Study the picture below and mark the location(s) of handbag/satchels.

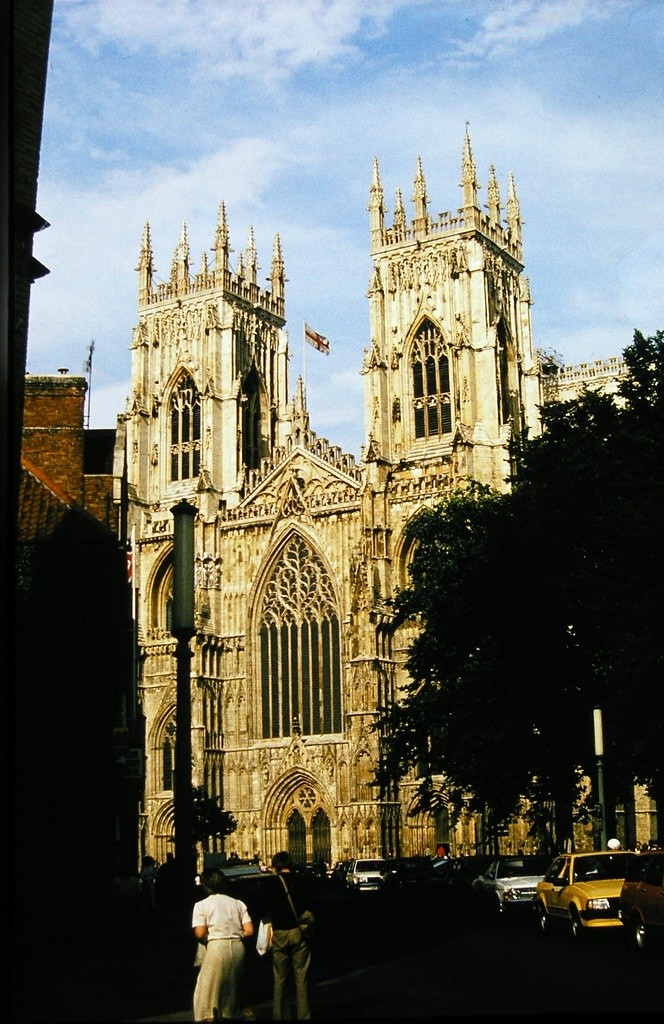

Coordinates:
[256,920,273,956]
[298,911,315,940]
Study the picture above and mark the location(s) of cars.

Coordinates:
[619,849,663,954]
[528,852,642,942]
[467,856,550,914]
[209,850,495,906]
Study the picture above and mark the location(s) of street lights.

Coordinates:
[169,497,198,887]
[592,705,608,855]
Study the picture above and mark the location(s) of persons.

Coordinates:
[261,850,319,1024]
[228,848,269,871]
[187,866,255,1024]
[436,845,446,858]
[422,844,433,858]
